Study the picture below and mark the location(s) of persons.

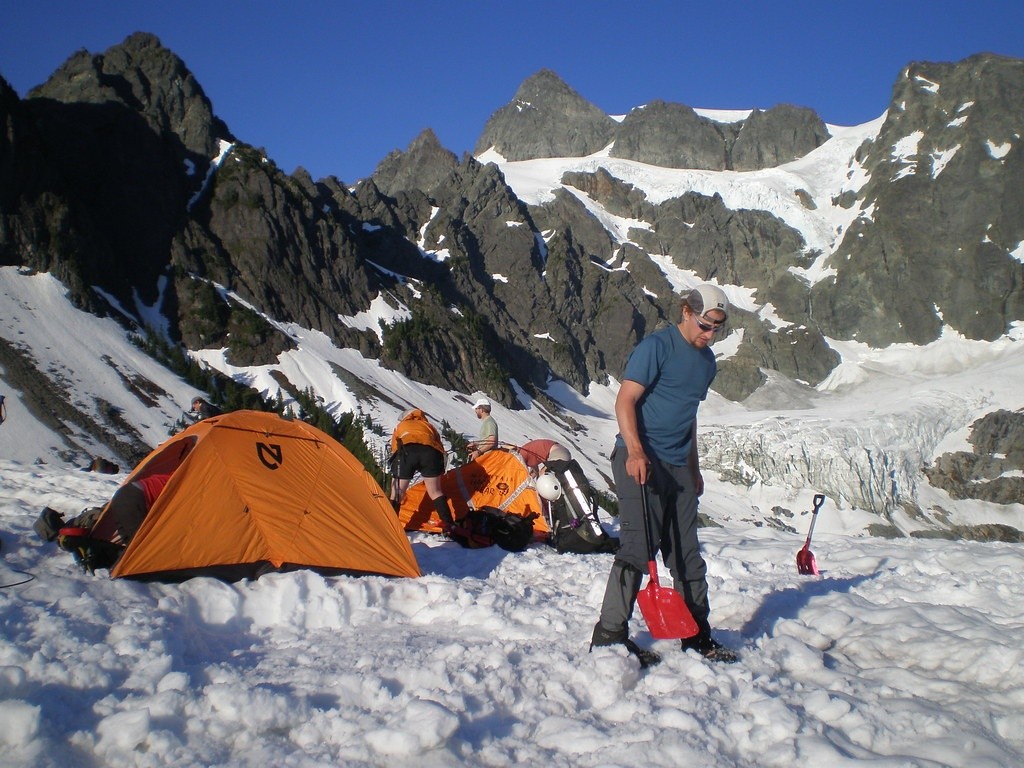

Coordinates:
[57,473,174,576]
[191,396,223,424]
[589,284,743,668]
[390,408,456,538]
[466,398,498,462]
[511,439,572,479]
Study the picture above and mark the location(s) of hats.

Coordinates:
[686,285,729,324]
[398,409,415,422]
[191,396,202,411]
[472,399,491,408]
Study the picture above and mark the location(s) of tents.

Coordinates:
[88,409,421,579]
[398,439,554,542]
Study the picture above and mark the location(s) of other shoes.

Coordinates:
[443,526,454,538]
[56,534,98,577]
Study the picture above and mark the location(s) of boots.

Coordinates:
[588,622,661,669]
[681,630,736,664]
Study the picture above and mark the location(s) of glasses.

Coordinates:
[691,312,720,332]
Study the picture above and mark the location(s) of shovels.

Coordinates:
[792,493,826,576]
[633,460,701,641]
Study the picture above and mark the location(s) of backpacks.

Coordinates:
[451,507,494,548]
[478,506,539,553]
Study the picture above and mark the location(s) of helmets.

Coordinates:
[536,471,562,502]
